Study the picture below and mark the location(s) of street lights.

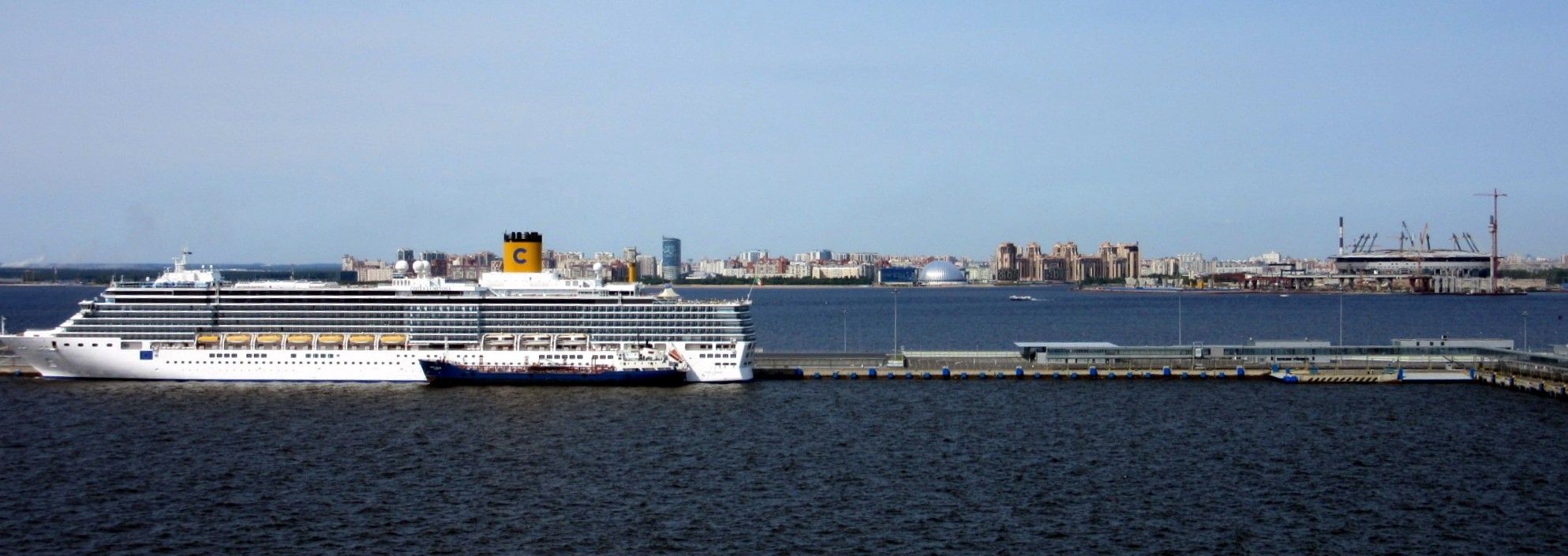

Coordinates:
[842,309,849,353]
[1336,288,1346,347]
[1522,310,1529,353]
[891,288,901,361]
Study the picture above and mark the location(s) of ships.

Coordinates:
[0,247,758,385]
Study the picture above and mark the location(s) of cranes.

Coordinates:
[1472,188,1508,280]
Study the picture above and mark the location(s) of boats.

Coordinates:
[350,335,374,344]
[657,287,680,301]
[416,357,688,388]
[226,334,250,344]
[317,334,343,344]
[288,335,313,344]
[1009,295,1031,301]
[197,336,219,343]
[258,335,282,344]
[382,335,406,346]
